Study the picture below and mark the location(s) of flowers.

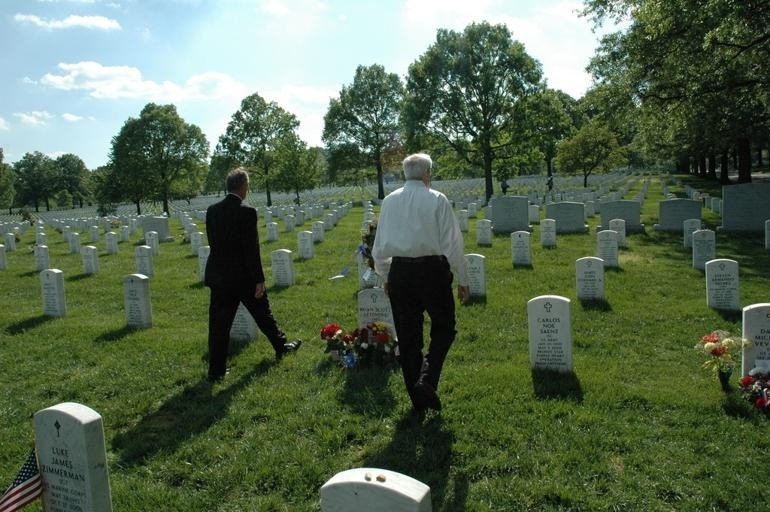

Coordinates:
[320,320,398,375]
[693,330,752,372]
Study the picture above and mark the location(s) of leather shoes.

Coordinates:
[206,367,224,384]
[275,339,301,362]
[412,377,442,412]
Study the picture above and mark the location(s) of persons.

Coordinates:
[204,168,302,382]
[371,153,471,412]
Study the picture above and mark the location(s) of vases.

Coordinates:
[717,369,732,388]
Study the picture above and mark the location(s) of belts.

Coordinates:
[391,254,445,263]
